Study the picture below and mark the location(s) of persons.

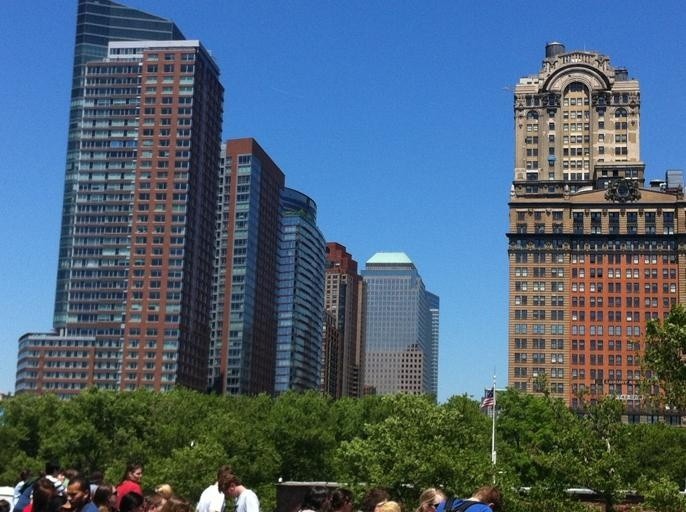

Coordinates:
[1,461,260,511]
[300,485,498,512]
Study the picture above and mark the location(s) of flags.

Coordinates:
[481,387,494,408]
